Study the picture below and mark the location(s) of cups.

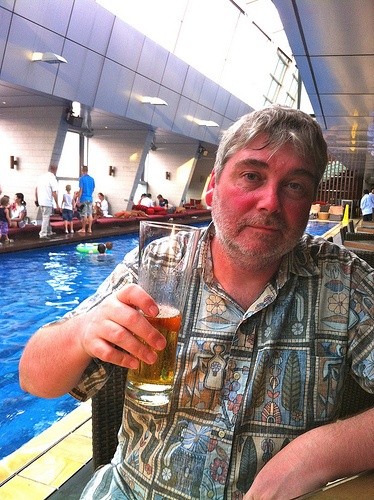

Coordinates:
[120,222,202,414]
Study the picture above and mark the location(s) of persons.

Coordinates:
[359,188,374,221]
[17,104,374,500]
[0,193,27,245]
[77,165,95,233]
[105,242,113,254]
[97,244,106,255]
[61,184,168,233]
[34,165,61,241]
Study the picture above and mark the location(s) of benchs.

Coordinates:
[0,208,220,238]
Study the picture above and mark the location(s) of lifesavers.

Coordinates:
[76,243,107,253]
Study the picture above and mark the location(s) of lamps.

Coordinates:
[166,171,171,180]
[10,156,17,169]
[198,144,209,161]
[66,102,86,128]
[33,51,68,66]
[142,95,169,106]
[207,121,217,128]
[109,165,114,176]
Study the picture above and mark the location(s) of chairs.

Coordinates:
[329,205,344,220]
[308,204,321,218]
[91,368,372,472]
[347,249,374,268]
[340,220,374,244]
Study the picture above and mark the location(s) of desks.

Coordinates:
[295,473,374,500]
[318,211,329,219]
[360,220,374,227]
[356,227,374,234]
[344,240,374,251]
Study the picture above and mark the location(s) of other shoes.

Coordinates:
[0,242,5,246]
[5,239,16,243]
[65,230,74,234]
[40,232,56,239]
[76,230,91,234]
